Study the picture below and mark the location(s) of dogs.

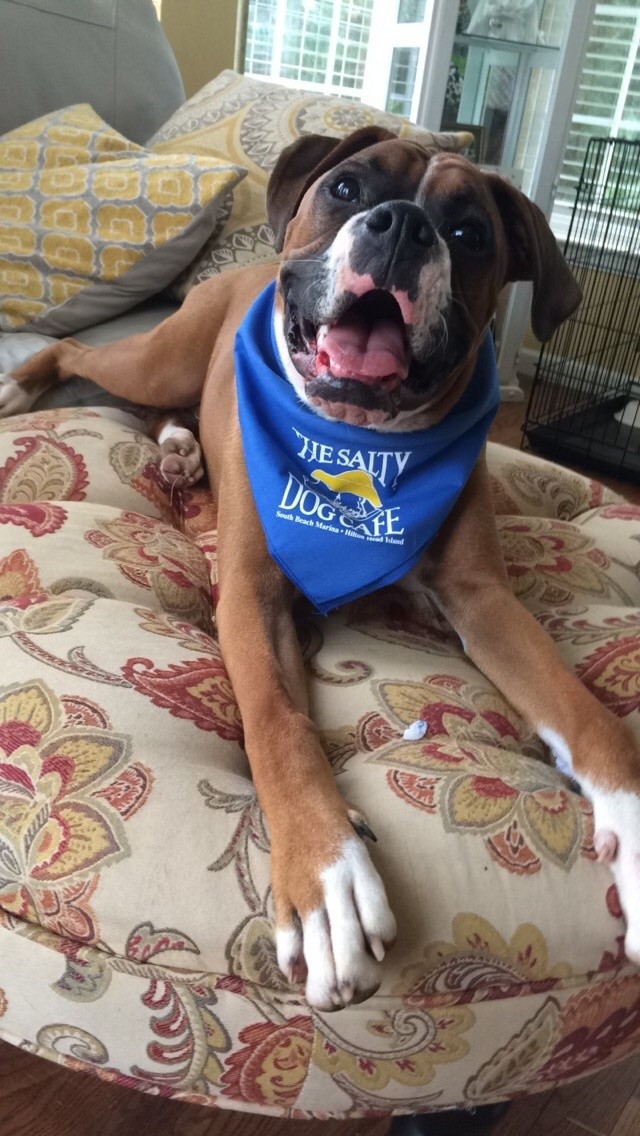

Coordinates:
[0,115,640,1018]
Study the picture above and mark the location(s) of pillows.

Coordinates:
[0,103,249,335]
[141,67,475,305]
